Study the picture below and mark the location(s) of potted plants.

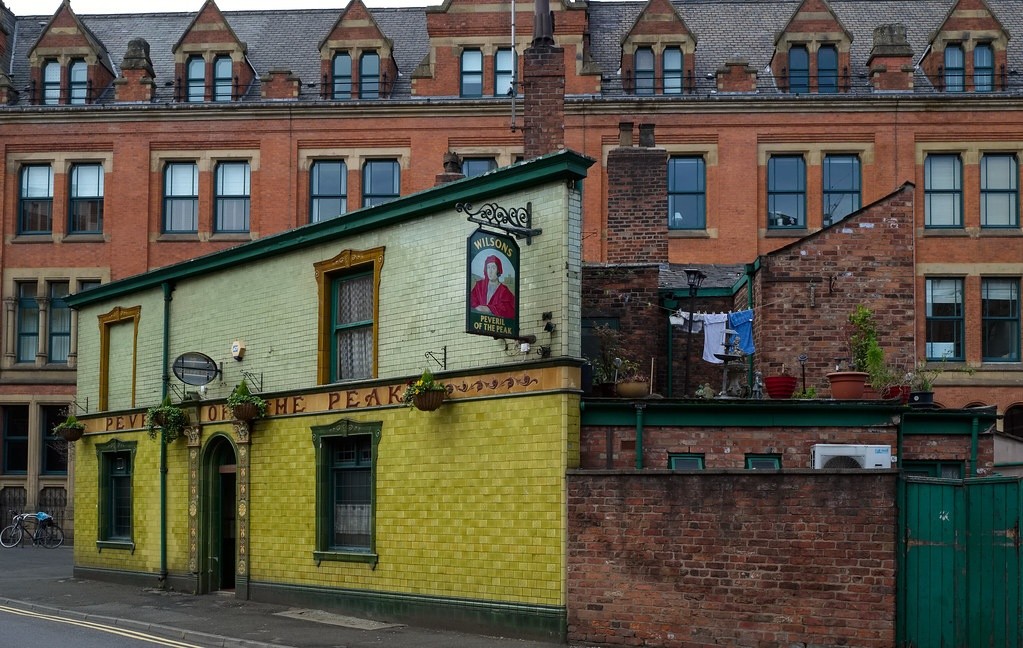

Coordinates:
[763,363,798,398]
[53,413,86,442]
[881,367,912,404]
[148,396,190,444]
[863,335,884,399]
[910,348,976,408]
[593,322,625,397]
[617,359,650,397]
[581,355,599,397]
[828,303,879,401]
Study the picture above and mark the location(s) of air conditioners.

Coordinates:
[810,442,891,469]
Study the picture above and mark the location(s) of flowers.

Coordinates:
[226,379,268,418]
[400,369,445,411]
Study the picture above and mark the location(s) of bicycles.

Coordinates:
[0,509,64,549]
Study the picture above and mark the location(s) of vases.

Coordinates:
[413,389,445,411]
[233,404,258,422]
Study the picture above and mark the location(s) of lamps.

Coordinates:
[672,211,683,227]
[694,274,707,291]
[684,268,699,289]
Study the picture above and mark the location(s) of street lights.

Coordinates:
[684,265,707,397]
